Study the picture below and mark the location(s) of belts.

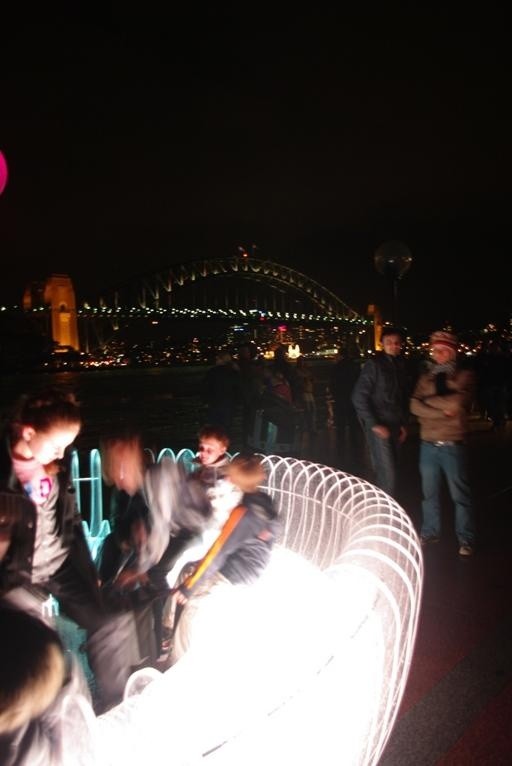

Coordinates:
[426,440,464,447]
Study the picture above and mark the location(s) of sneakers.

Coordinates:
[419,535,441,545]
[458,542,472,557]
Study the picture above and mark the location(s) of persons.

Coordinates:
[0,385,276,765]
[353,324,415,496]
[196,346,365,462]
[409,329,479,558]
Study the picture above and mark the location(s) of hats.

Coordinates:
[429,327,460,359]
